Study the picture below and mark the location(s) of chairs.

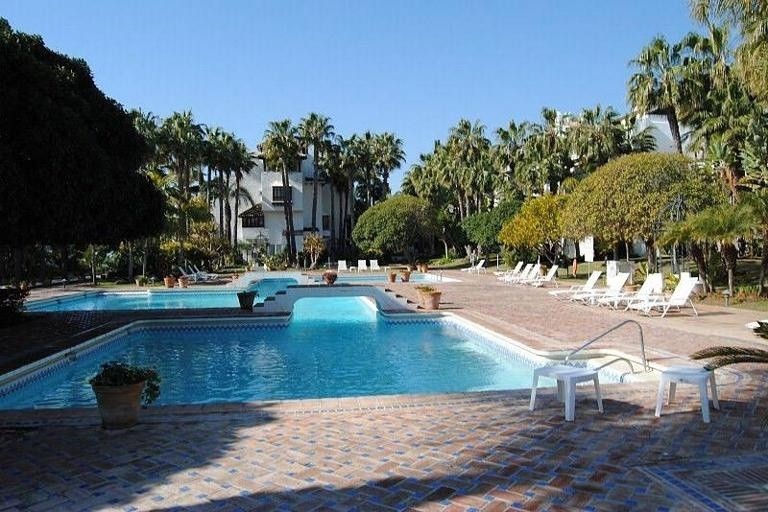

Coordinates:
[549,270,700,319]
[468,259,486,275]
[337,259,348,274]
[357,259,367,274]
[370,259,380,272]
[178,263,220,284]
[493,261,559,289]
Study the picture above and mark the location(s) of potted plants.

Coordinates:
[387,272,396,282]
[400,270,411,281]
[135,276,148,287]
[88,357,162,428]
[415,283,441,310]
[237,290,259,312]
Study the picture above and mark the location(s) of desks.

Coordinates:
[350,266,356,272]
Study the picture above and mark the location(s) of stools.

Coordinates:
[655,366,720,425]
[529,365,605,421]
